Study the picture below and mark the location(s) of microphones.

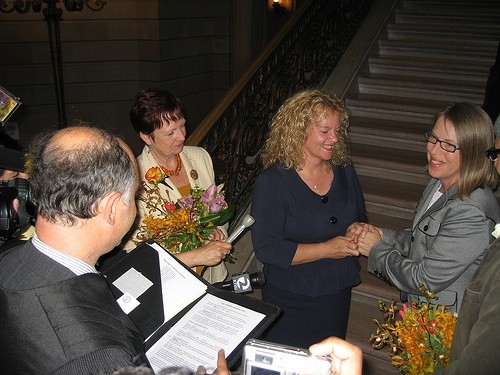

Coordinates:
[212,272,266,294]
[226,215,255,244]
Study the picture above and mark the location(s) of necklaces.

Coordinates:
[152,153,182,176]
[300,168,329,193]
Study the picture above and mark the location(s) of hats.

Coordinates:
[0,133,26,172]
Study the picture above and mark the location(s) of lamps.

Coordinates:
[272,0,286,17]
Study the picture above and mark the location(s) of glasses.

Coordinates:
[485,147,500,162]
[424,131,459,152]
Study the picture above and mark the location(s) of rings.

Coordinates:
[224,249,228,254]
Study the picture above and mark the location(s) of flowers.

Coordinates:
[129,166,237,265]
[368,284,457,375]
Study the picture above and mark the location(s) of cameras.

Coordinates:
[242,339,332,375]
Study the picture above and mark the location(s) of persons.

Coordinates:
[432,116,500,375]
[0,88,233,375]
[252,89,368,350]
[346,103,495,366]
[309,337,363,375]
